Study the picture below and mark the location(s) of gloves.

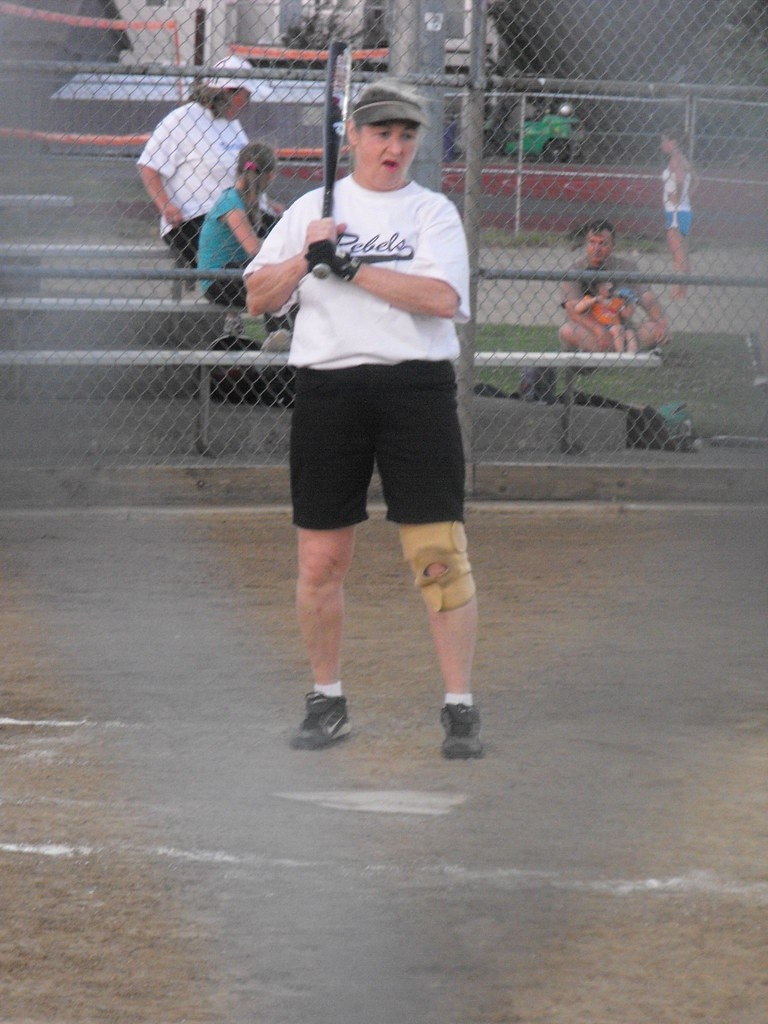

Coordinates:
[305,239,362,282]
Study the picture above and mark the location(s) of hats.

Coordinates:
[350,80,434,131]
[202,55,256,93]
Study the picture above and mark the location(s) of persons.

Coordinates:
[136,53,287,269]
[573,282,640,354]
[197,140,300,352]
[661,128,701,304]
[558,220,670,354]
[243,83,485,758]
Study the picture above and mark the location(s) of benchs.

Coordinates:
[0,194,661,460]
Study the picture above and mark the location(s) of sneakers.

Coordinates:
[291,692,352,750]
[440,703,485,759]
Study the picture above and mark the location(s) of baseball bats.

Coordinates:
[312,38,352,281]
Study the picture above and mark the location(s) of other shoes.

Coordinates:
[225,318,245,334]
[259,329,291,352]
